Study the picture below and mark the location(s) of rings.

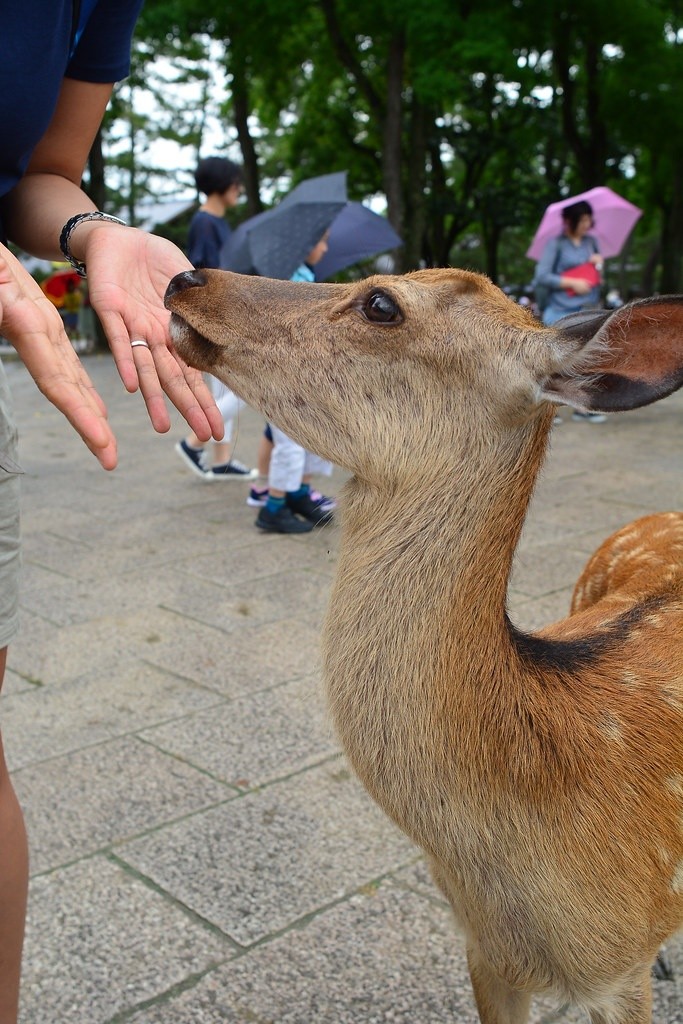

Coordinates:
[130,341,148,347]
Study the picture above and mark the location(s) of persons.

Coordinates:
[519,286,539,319]
[0,0,223,1024]
[534,201,607,423]
[247,422,336,511]
[175,156,258,480]
[605,289,623,310]
[253,228,332,535]
[63,279,81,352]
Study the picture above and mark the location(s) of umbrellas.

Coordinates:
[525,186,641,262]
[222,200,402,282]
[220,172,347,282]
[39,269,82,308]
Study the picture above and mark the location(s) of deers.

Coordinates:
[163,267,683,1024]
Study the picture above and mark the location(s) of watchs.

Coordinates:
[594,263,603,270]
[59,211,126,278]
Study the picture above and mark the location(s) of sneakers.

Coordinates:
[212,459,260,480]
[290,495,334,526]
[310,491,337,511]
[256,506,313,533]
[573,411,606,423]
[174,439,213,481]
[554,415,563,424]
[246,485,270,507]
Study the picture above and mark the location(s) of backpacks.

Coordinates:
[533,235,599,312]
